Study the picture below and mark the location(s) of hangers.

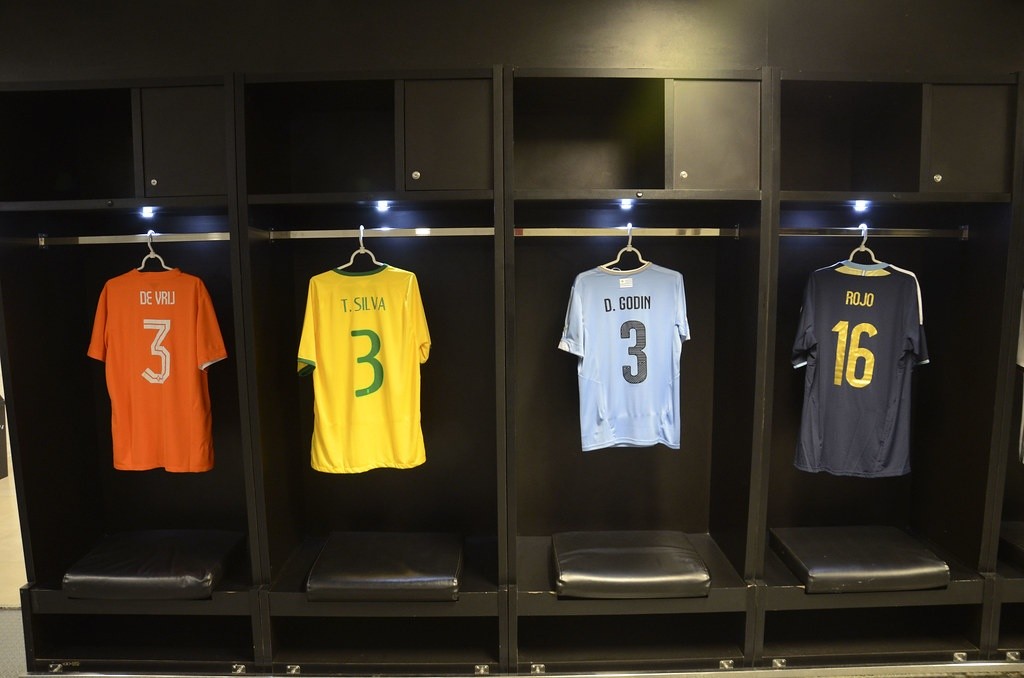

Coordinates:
[135,229,175,275]
[599,221,652,276]
[335,224,387,274]
[842,223,889,266]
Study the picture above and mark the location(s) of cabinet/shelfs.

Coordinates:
[6,70,1022,675]
